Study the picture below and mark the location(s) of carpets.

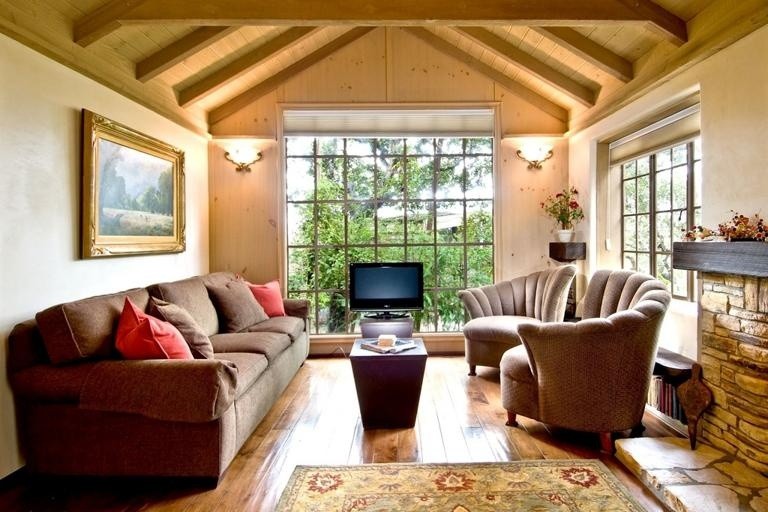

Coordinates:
[275,458,652,512]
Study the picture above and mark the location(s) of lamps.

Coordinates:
[213,137,272,173]
[505,135,561,171]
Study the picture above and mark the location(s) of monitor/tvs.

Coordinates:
[350,262,424,319]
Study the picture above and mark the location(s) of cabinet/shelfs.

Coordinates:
[360,317,412,336]
[644,347,697,440]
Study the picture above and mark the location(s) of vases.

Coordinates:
[731,237,756,242]
[556,230,574,242]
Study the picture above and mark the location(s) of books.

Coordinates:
[647,371,689,424]
[360,332,414,354]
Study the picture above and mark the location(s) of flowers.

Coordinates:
[677,211,768,245]
[539,186,585,230]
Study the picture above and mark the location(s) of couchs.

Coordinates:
[499,269,672,457]
[457,265,578,376]
[6,271,313,489]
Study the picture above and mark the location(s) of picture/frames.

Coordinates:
[78,108,186,261]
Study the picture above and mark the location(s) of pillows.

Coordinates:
[113,297,194,361]
[204,280,270,332]
[248,280,285,318]
[145,299,214,361]
